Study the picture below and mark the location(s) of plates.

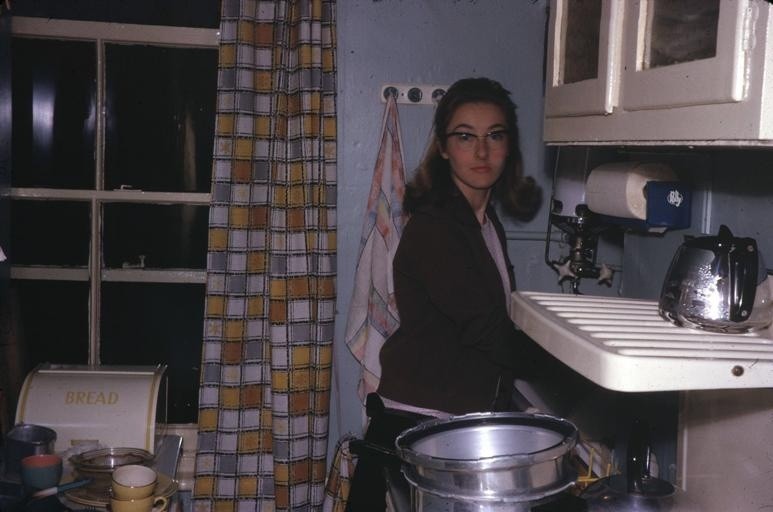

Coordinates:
[64,471,181,506]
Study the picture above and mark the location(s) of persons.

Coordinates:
[342,77,547,511]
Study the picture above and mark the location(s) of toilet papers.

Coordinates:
[584,161,677,220]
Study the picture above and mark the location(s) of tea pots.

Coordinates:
[572,416,700,512]
[657,226,772,335]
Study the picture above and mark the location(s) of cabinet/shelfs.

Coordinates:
[542,0,773,147]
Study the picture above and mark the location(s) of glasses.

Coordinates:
[446,129,516,150]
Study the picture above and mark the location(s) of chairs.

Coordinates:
[15,362,183,480]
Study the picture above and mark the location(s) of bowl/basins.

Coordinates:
[66,445,155,494]
[19,453,61,486]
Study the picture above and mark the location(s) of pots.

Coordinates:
[393,410,582,512]
[2,423,58,469]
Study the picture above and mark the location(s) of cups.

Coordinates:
[110,463,157,501]
[108,492,167,511]
